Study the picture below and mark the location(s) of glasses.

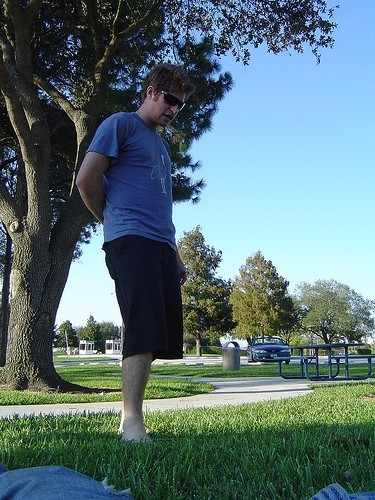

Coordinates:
[160,90,185,111]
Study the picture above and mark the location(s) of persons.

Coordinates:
[76,63,197,444]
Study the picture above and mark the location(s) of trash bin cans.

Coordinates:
[221,341,240,372]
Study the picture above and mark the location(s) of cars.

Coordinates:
[246,335,290,364]
